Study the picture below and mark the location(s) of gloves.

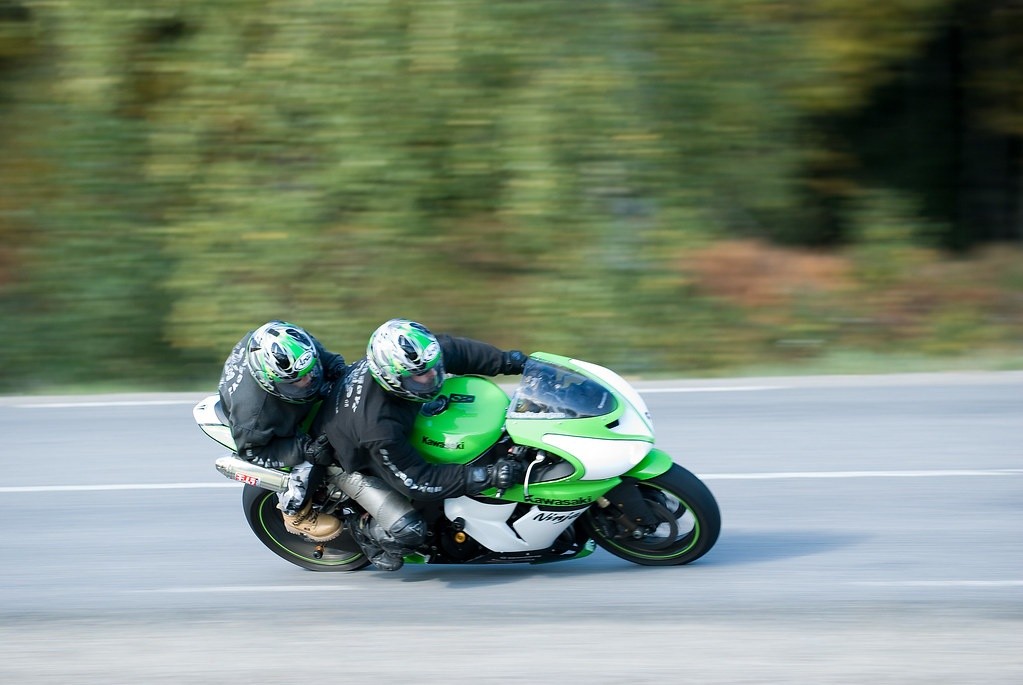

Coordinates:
[345,361,357,373]
[466,461,521,494]
[302,433,334,466]
[502,350,557,381]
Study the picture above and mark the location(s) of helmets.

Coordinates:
[246,319,324,406]
[366,318,445,403]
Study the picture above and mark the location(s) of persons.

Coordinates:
[218,320,349,544]
[323,318,528,571]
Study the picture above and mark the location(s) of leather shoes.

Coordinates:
[276,501,344,542]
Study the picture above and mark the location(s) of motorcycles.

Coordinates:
[193,350,721,573]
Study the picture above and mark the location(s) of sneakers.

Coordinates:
[345,513,405,571]
